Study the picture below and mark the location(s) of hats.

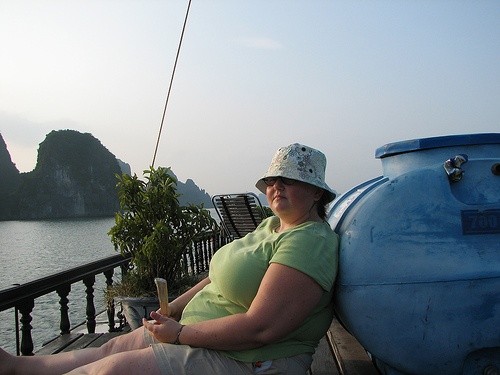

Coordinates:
[254,143,337,206]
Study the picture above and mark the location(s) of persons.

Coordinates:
[0,142,340,375]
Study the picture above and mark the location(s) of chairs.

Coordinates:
[210,193,268,238]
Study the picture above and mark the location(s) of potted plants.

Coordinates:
[103,166,221,329]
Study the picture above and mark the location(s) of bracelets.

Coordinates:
[175,324,185,345]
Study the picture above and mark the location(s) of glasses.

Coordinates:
[262,177,297,186]
